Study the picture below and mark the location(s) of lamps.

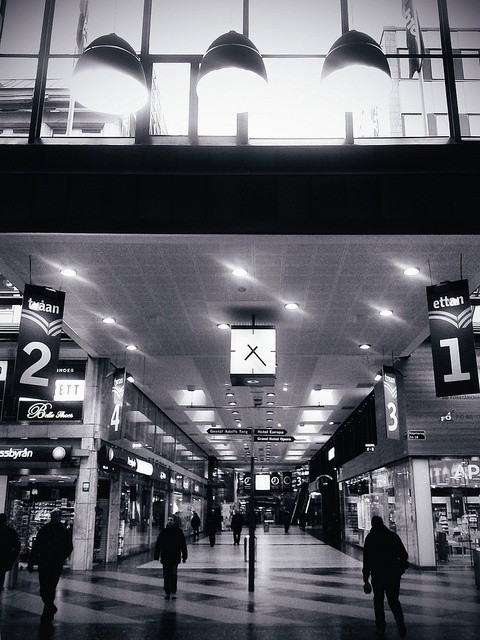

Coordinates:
[319,29,392,114]
[69,33,149,115]
[374,371,384,381]
[194,30,269,114]
[109,361,135,385]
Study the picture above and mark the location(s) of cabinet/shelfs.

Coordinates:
[9,499,31,558]
[28,499,75,552]
[461,496,480,532]
[431,496,452,541]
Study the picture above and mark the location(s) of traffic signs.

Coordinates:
[254,436,296,442]
[207,428,251,434]
[254,429,287,435]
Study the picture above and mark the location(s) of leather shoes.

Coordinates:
[165,593,170,600]
[172,593,176,600]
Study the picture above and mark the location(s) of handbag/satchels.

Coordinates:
[364,581,371,594]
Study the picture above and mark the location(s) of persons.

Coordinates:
[1,513,21,595]
[362,515,408,637]
[27,509,73,628]
[283,510,290,533]
[205,513,218,547]
[175,512,181,528]
[154,515,187,599]
[231,510,243,545]
[191,513,201,542]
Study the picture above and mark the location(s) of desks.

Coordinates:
[453,538,473,559]
